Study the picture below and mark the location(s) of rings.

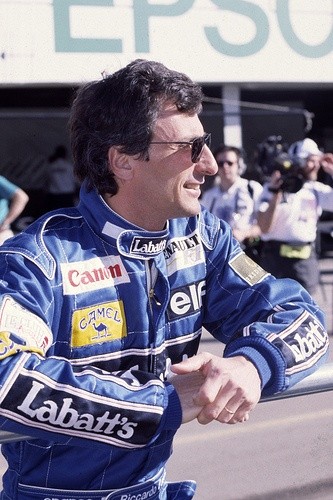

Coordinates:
[225,407,235,415]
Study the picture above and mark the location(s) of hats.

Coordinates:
[289,138,321,169]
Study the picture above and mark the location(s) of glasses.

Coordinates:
[217,160,239,168]
[148,133,211,163]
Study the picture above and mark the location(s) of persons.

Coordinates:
[38,146,81,208]
[0,58,329,500]
[0,176,29,245]
[200,145,263,251]
[251,139,333,295]
